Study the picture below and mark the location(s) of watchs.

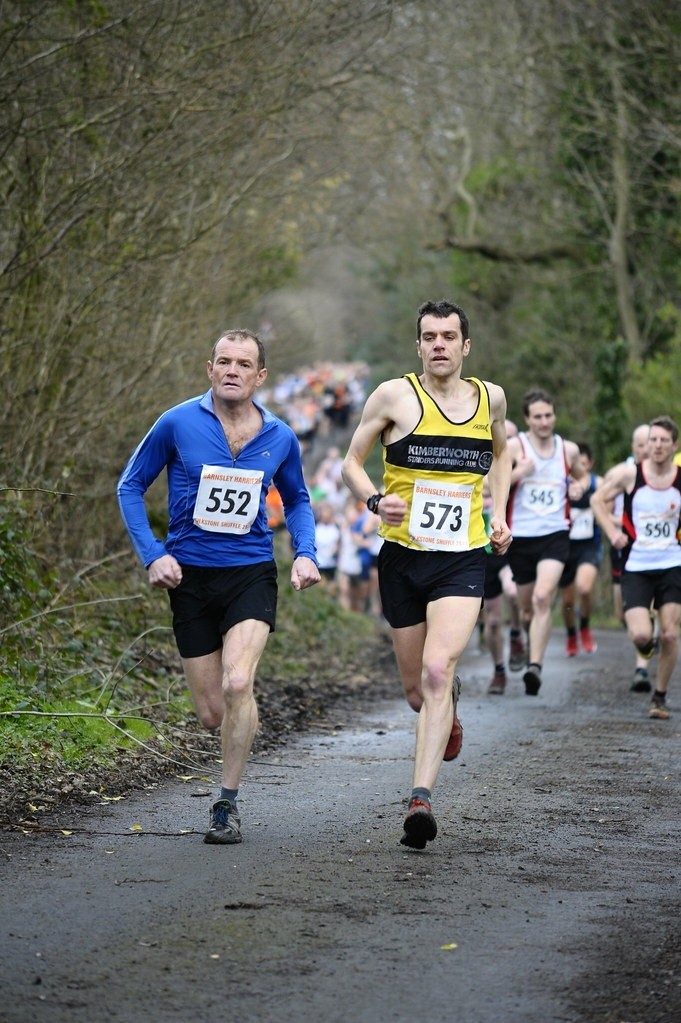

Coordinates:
[367,494,385,515]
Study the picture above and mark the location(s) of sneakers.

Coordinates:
[400,796,438,849]
[440,675,463,761]
[205,798,241,845]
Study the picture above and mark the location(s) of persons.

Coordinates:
[477,389,681,718]
[116,329,322,844]
[257,360,370,472]
[341,300,512,850]
[266,443,384,617]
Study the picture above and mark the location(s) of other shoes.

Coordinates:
[650,701,669,719]
[567,631,579,655]
[523,671,541,695]
[489,673,506,694]
[580,628,597,655]
[631,668,651,692]
[508,632,526,671]
